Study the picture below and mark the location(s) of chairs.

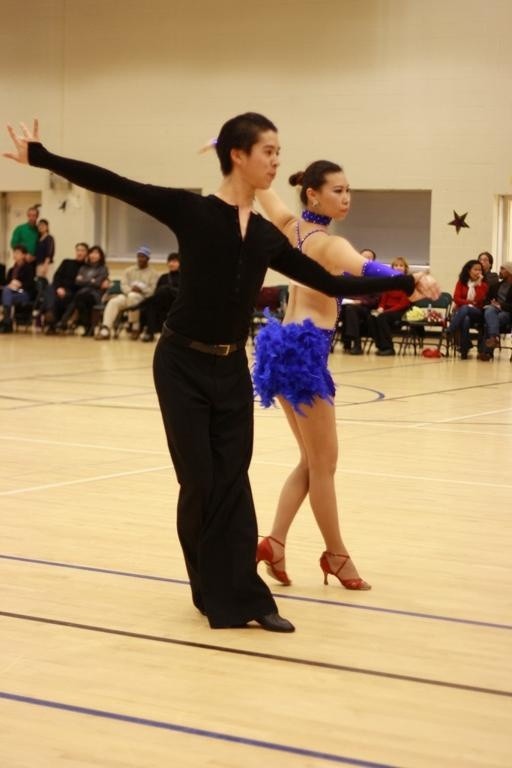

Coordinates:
[0,265,512,361]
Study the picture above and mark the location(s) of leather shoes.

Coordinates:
[257,613,296,633]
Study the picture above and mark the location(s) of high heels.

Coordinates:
[319,552,368,590]
[256,535,292,586]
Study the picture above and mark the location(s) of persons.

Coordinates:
[0,208,110,337]
[193,130,441,592]
[98,247,181,341]
[0,111,425,634]
[341,250,448,355]
[447,252,512,363]
